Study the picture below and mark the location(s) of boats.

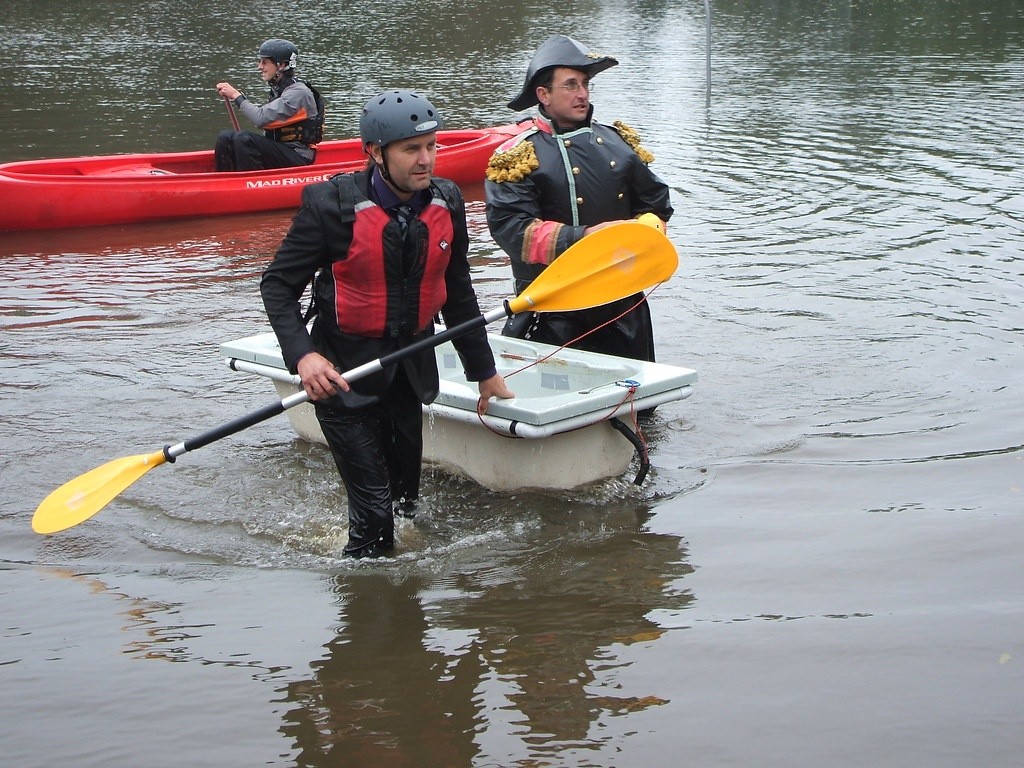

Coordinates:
[0,115,539,234]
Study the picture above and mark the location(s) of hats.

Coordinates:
[506,33,619,112]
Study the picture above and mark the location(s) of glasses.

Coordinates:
[545,81,595,92]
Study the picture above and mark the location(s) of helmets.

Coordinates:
[257,39,298,64]
[360,90,442,153]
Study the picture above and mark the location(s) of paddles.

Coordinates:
[31,222,678,534]
[223,95,240,132]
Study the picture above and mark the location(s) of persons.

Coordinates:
[215,39,325,172]
[484,34,674,364]
[261,90,515,558]
